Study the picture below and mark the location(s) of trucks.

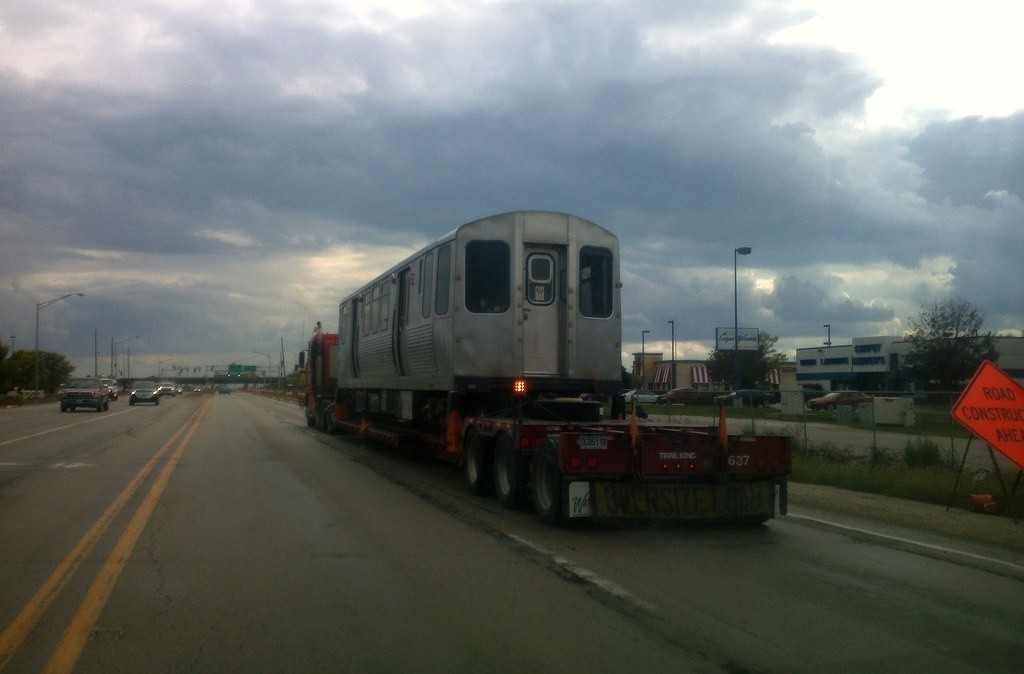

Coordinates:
[296,335,794,532]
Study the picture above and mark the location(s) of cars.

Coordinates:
[157,382,176,398]
[714,389,777,410]
[806,390,874,413]
[658,387,699,405]
[174,384,183,396]
[621,390,659,403]
[100,379,120,401]
[192,386,201,392]
[129,381,159,407]
[59,377,109,411]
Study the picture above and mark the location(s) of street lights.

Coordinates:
[115,335,138,379]
[733,246,752,389]
[32,293,84,405]
